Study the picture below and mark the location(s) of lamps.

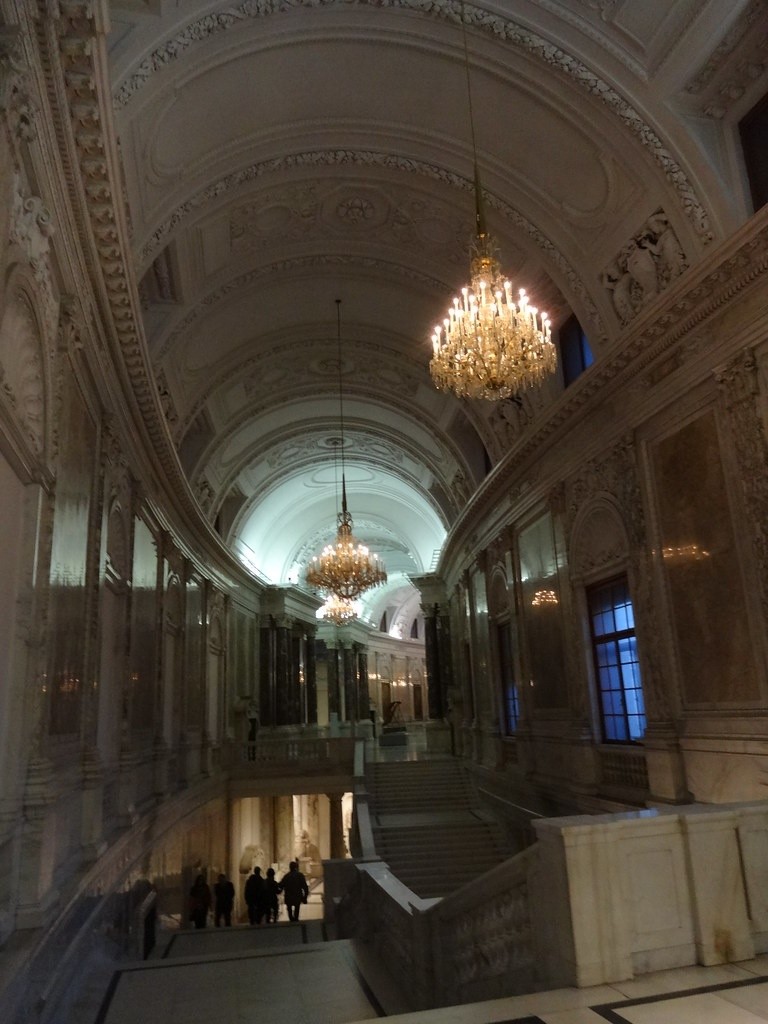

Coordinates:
[303,300,390,627]
[428,1,556,400]
[530,524,558,608]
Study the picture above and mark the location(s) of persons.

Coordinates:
[369,697,376,724]
[244,866,284,925]
[213,874,234,927]
[189,875,212,929]
[489,390,535,454]
[279,862,308,921]
[602,215,688,321]
